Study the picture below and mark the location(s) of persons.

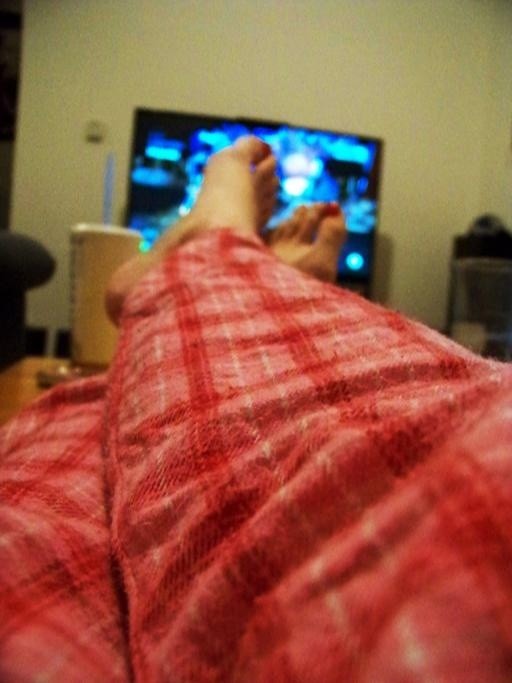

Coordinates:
[1,132,511,681]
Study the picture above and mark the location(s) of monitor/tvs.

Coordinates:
[123,106,383,284]
